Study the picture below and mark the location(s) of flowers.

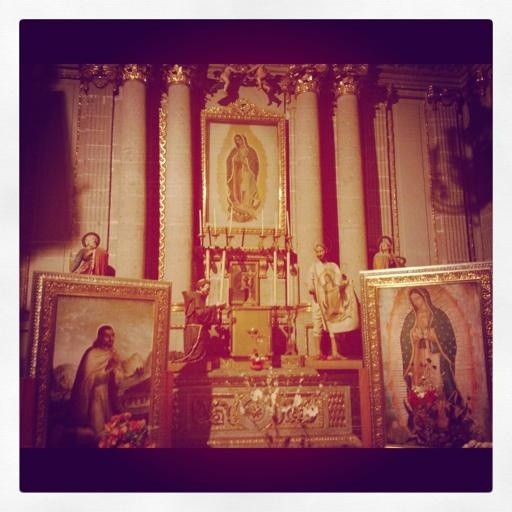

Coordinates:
[99,414,157,448]
[404,373,474,449]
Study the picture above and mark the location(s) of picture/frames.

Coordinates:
[19,270,172,448]
[201,97,287,235]
[360,261,493,449]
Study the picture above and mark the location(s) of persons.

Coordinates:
[68,325,125,440]
[70,232,107,277]
[309,242,344,361]
[234,264,254,290]
[322,272,343,318]
[400,286,464,415]
[182,279,227,364]
[372,236,399,271]
[226,132,259,223]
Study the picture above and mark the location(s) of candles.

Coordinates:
[220,251,225,302]
[205,250,209,306]
[261,210,264,235]
[274,211,278,236]
[213,208,216,233]
[274,251,277,305]
[230,206,232,235]
[199,211,202,235]
[287,252,290,306]
